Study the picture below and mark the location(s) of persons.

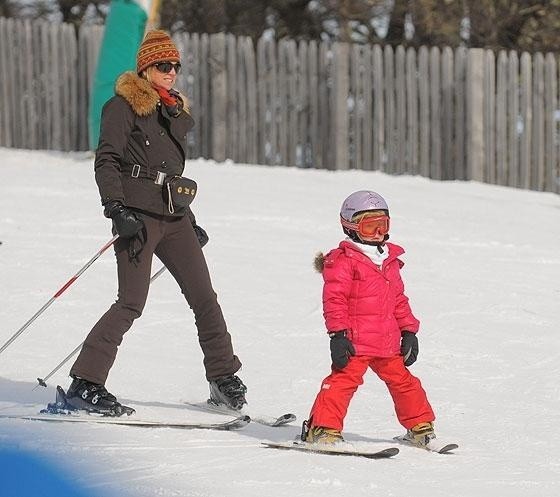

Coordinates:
[64,30,248,412]
[301,191,446,452]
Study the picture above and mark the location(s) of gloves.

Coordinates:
[103,200,143,240]
[330,330,356,366]
[193,225,209,249]
[399,330,419,366]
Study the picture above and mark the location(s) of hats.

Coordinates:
[136,30,181,74]
[340,190,390,234]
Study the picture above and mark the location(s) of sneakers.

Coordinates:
[210,374,248,411]
[306,425,344,447]
[408,422,436,444]
[54,378,136,417]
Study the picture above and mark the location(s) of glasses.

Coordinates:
[155,62,181,74]
[358,216,391,237]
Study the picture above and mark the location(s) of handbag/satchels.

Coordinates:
[163,175,197,207]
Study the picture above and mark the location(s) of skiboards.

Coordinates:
[1,394,295,430]
[262,432,457,458]
